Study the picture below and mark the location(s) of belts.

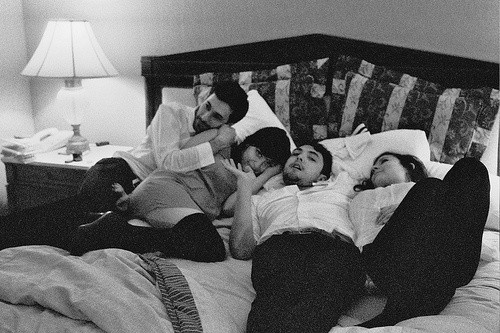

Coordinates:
[284,227,352,244]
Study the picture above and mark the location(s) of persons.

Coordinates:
[221,141,357,333]
[71,127,291,263]
[76,80,250,224]
[347,153,491,328]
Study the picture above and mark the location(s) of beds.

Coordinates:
[0,34,500,333]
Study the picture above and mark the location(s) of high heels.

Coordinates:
[66,211,123,247]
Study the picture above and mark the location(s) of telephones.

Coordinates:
[29,127,74,156]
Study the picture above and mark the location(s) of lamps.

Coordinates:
[21,19,121,159]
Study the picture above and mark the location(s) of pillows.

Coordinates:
[193,57,332,144]
[315,129,431,177]
[329,55,500,163]
[431,161,500,231]
[232,89,298,157]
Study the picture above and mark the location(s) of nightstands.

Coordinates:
[6,144,137,213]
